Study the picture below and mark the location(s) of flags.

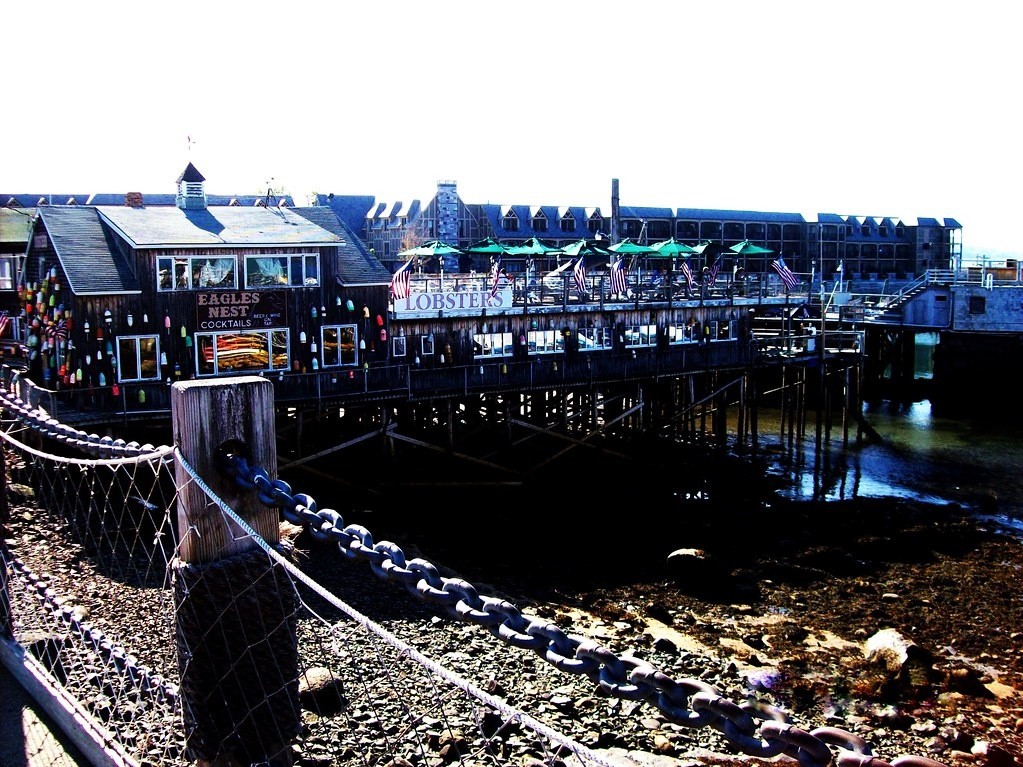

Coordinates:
[680,263,694,289]
[490,254,504,298]
[771,256,796,291]
[608,259,626,295]
[391,257,416,299]
[574,255,587,292]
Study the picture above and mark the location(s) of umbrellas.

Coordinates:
[395,239,464,272]
[463,238,513,271]
[604,238,660,268]
[694,240,736,271]
[730,238,775,270]
[649,235,696,270]
[505,236,566,272]
[560,237,610,275]
